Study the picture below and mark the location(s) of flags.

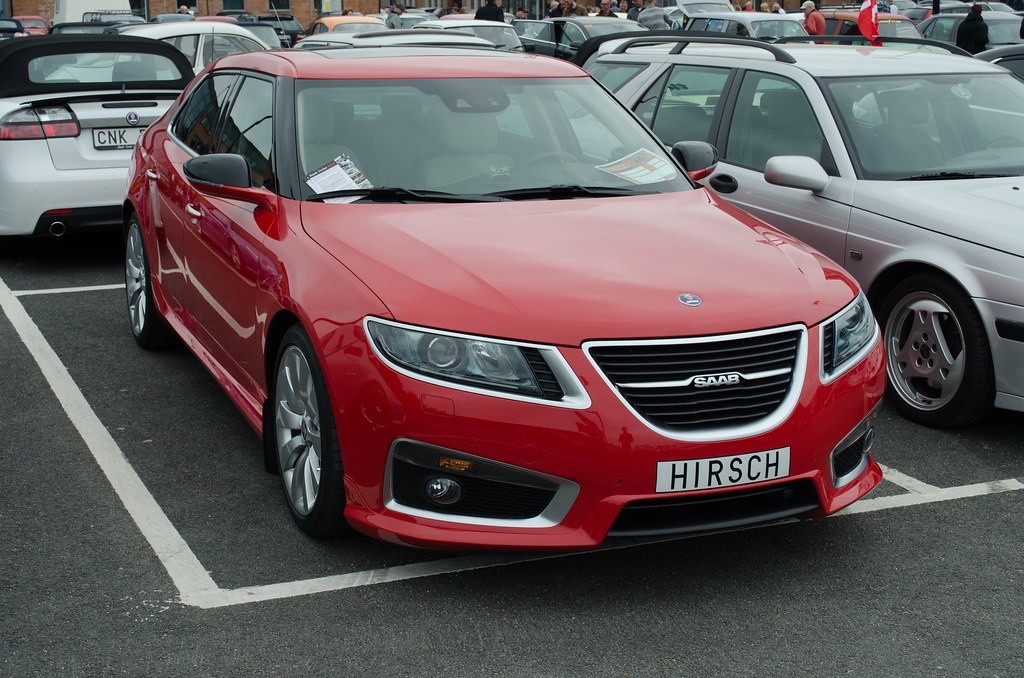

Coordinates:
[856,0,882,46]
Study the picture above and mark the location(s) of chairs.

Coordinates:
[331,101,374,179]
[653,106,707,146]
[190,35,213,67]
[870,93,944,169]
[373,96,442,188]
[417,106,517,189]
[302,111,366,189]
[111,61,157,81]
[745,91,821,169]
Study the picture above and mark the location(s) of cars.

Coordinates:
[120,41,885,557]
[566,38,1024,433]
[0,0,1024,242]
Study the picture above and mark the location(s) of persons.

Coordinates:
[800,1,826,48]
[385,4,408,32]
[474,0,788,27]
[341,9,354,18]
[1020,10,1024,42]
[448,6,469,17]
[864,0,901,16]
[957,4,989,55]
[178,6,191,16]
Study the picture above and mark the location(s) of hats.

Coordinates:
[800,1,815,9]
[395,4,405,13]
